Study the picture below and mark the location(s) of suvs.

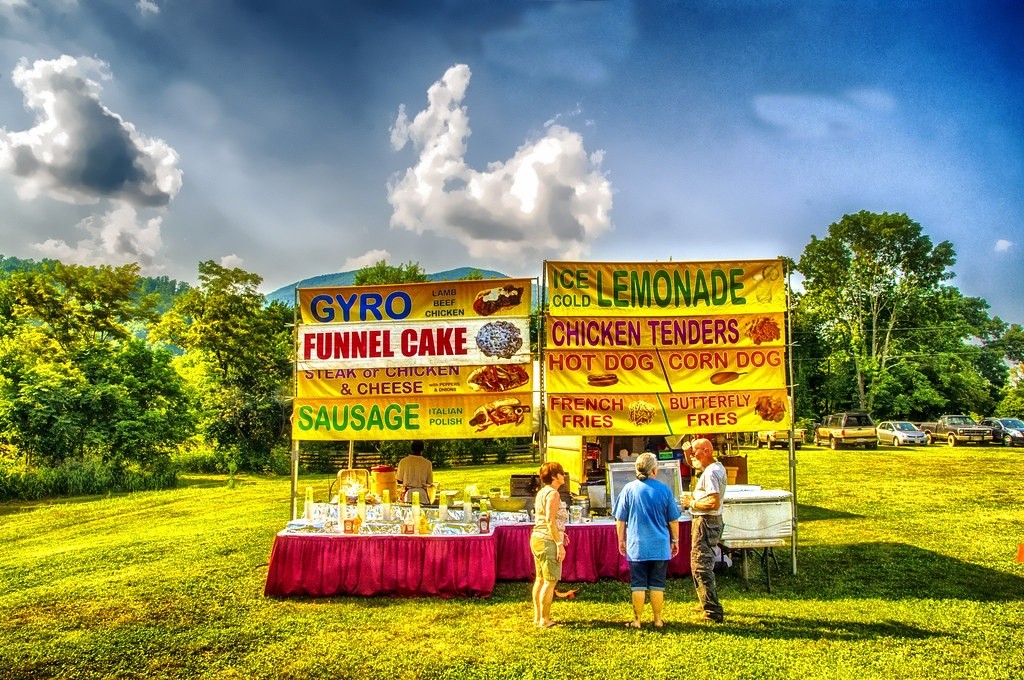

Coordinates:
[978,419,1024,448]
[812,412,877,451]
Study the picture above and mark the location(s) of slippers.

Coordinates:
[625,621,641,630]
[653,620,665,628]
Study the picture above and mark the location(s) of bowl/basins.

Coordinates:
[490,497,527,511]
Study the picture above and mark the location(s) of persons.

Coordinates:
[530,462,570,629]
[680,438,727,623]
[395,440,433,505]
[613,452,681,630]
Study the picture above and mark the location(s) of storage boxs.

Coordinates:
[719,491,792,541]
[508,427,768,502]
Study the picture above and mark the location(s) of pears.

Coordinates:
[710,372,748,385]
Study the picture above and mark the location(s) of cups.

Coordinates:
[570,506,582,524]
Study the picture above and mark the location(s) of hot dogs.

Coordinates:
[587,374,619,386]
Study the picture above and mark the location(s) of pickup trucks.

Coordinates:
[920,415,994,447]
[757,429,804,450]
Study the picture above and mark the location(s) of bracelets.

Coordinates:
[672,539,679,542]
[690,500,697,510]
[556,541,563,546]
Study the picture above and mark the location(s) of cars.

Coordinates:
[876,421,929,447]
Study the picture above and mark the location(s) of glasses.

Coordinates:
[690,447,705,456]
[556,471,564,476]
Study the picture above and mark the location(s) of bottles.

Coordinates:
[404,512,414,534]
[344,514,353,534]
[353,514,362,534]
[479,513,489,534]
[419,514,427,534]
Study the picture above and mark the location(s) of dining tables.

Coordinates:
[267,503,693,598]
